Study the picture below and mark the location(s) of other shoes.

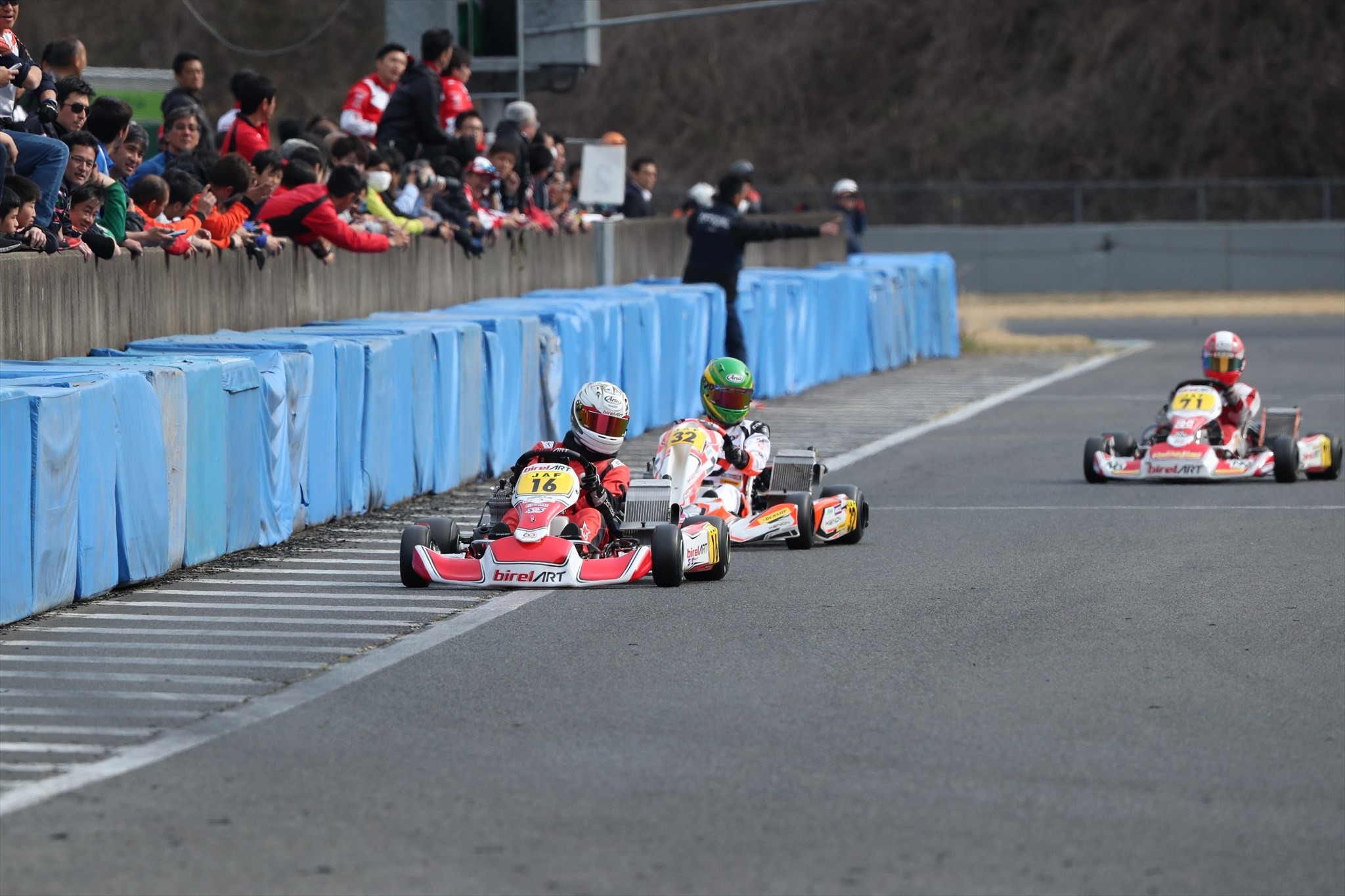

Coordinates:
[487,521,511,540]
[699,490,718,516]
[1207,419,1223,446]
[57,236,82,251]
[560,521,580,554]
[0,238,22,253]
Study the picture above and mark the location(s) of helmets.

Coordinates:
[832,178,858,197]
[1202,330,1245,387]
[700,357,753,425]
[570,382,629,454]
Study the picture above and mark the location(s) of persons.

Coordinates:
[489,381,630,557]
[1155,330,1261,458]
[682,172,841,364]
[832,177,866,253]
[0,0,762,270]
[697,356,770,518]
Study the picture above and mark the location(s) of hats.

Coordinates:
[462,156,502,181]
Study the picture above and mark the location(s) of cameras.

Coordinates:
[451,227,485,257]
[426,173,463,190]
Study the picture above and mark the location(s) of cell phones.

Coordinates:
[253,228,263,236]
[8,63,22,70]
[167,229,188,236]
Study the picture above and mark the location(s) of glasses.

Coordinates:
[481,164,496,174]
[0,0,19,6]
[70,156,94,168]
[58,101,91,117]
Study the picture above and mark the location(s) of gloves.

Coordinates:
[580,463,601,494]
[725,444,743,466]
[509,466,517,486]
[1222,387,1240,406]
[39,98,58,124]
